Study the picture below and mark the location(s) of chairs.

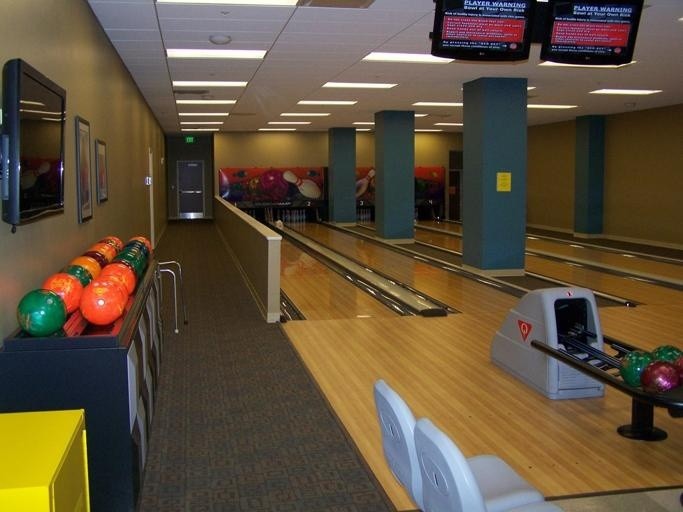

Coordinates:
[370,378,565,512]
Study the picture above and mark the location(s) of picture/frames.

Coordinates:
[73,114,109,226]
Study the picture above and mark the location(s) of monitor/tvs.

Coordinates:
[431,0,538,62]
[540,0,645,66]
[0,57,67,226]
